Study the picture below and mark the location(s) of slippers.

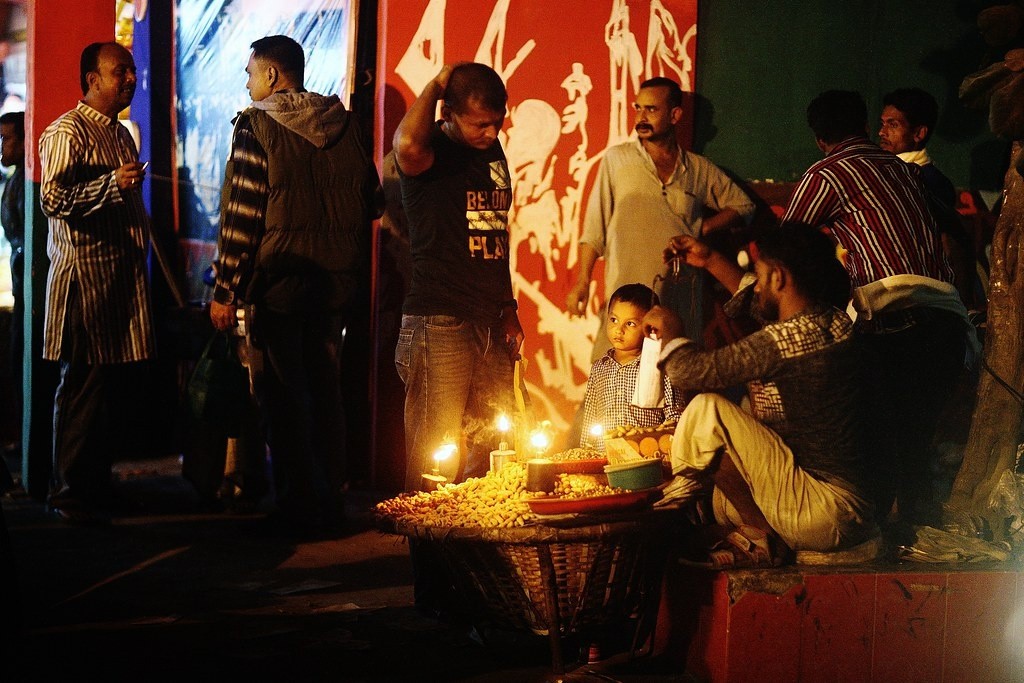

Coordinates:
[678,539,777,569]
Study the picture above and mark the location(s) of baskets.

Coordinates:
[375,489,645,646]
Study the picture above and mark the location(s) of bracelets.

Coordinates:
[500,299,518,310]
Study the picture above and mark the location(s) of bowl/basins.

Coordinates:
[603,458,662,490]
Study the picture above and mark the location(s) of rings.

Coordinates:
[131,178,135,184]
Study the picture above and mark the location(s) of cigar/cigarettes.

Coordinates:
[141,161,149,171]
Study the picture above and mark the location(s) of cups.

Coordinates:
[527,459,555,494]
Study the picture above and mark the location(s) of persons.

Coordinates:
[37,41,159,524]
[578,282,688,454]
[565,78,757,365]
[777,90,985,559]
[209,35,387,548]
[391,62,525,620]
[651,222,900,576]
[874,90,968,309]
[0,111,25,471]
